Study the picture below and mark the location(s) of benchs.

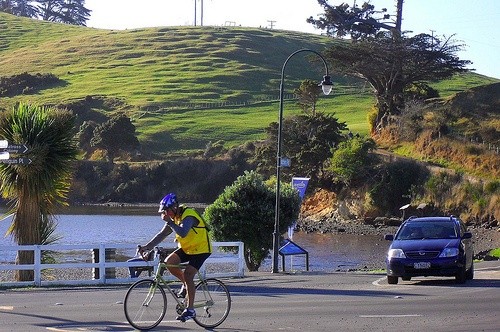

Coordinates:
[133,247,176,276]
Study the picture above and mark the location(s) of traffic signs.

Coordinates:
[0,140,32,166]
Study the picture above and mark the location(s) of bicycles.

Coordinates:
[123,244,231,331]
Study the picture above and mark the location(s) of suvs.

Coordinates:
[384,214,474,284]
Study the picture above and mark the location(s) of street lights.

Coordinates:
[270,49,334,274]
[429,30,436,50]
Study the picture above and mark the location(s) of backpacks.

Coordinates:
[181,205,211,233]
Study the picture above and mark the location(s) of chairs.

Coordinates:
[410,227,424,236]
[440,226,452,236]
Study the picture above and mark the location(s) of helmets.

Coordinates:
[157,192,179,216]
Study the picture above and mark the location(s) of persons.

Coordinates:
[138,193,212,320]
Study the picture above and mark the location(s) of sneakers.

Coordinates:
[176,308,196,320]
[174,285,186,300]
